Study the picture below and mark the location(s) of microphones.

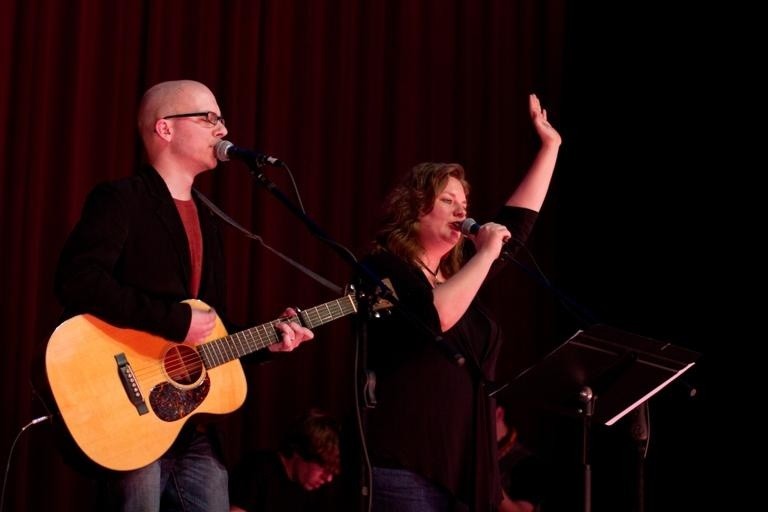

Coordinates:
[460,218,520,248]
[214,140,282,166]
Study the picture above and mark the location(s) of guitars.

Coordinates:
[32,278,398,472]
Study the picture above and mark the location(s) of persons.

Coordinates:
[51,80,315,512]
[350,93,562,512]
[227,410,345,512]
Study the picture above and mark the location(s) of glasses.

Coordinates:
[154,112,228,127]
